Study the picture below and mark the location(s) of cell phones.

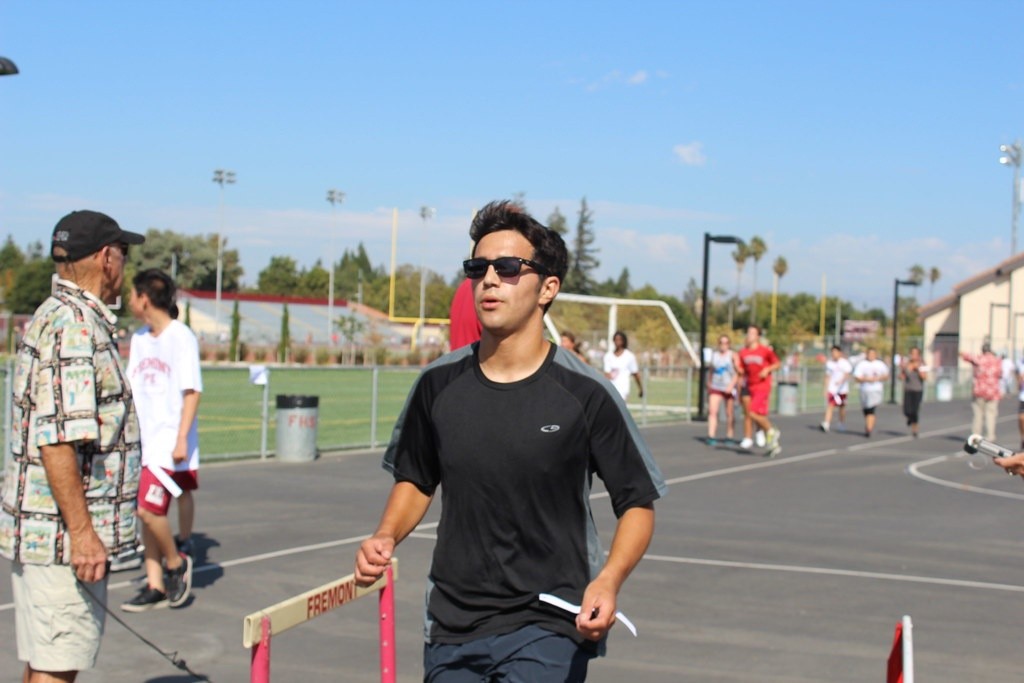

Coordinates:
[967,435,1015,459]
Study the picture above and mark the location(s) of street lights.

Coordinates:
[889,277,919,403]
[326,189,346,342]
[690,232,742,421]
[209,167,237,336]
[419,204,436,318]
[1000,138,1023,257]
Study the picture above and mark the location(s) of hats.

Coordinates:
[50,209,146,263]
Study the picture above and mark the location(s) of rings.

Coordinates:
[1009,469,1013,476]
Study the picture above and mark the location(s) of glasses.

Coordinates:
[107,242,129,256]
[463,257,554,279]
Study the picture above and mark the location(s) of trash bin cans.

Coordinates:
[276,393,319,460]
[777,381,797,415]
[935,375,953,402]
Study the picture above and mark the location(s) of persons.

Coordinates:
[604,331,644,403]
[852,347,890,436]
[819,345,853,432]
[354,200,665,683]
[122,267,203,612]
[956,343,1001,442]
[898,347,927,437]
[583,342,850,376]
[736,325,782,458]
[1015,354,1024,450]
[561,331,589,369]
[706,335,739,446]
[0,210,147,683]
[449,205,527,354]
[990,451,1024,483]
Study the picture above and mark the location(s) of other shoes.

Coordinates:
[839,424,846,432]
[766,428,780,449]
[755,429,766,448]
[820,421,830,433]
[770,447,782,458]
[706,438,717,447]
[907,419,911,426]
[108,544,141,571]
[914,432,919,439]
[865,428,872,437]
[175,536,198,565]
[725,439,737,447]
[739,438,754,449]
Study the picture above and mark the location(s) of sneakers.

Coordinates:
[167,552,193,607]
[120,583,170,613]
